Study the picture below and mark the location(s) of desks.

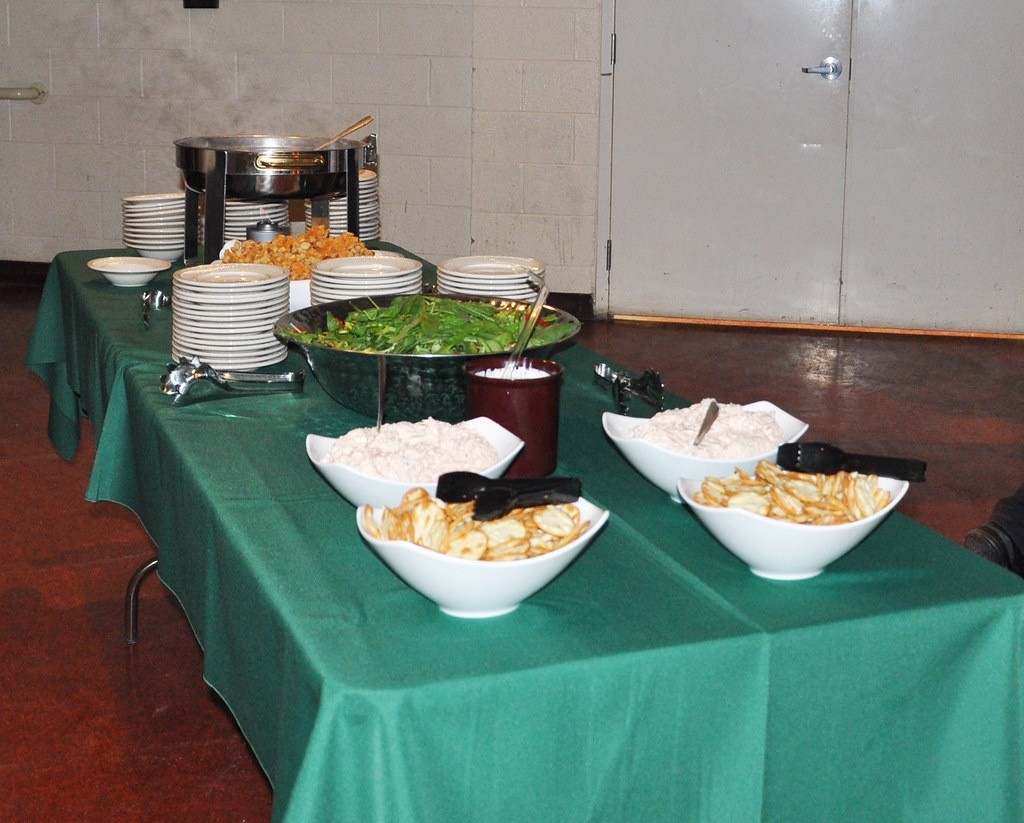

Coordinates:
[28,249,1024,823]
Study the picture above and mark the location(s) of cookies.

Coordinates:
[363,485,590,562]
[695,458,891,525]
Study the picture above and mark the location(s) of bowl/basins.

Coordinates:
[121,194,186,262]
[86,255,171,288]
[273,295,583,423]
[355,501,610,618]
[601,401,809,502]
[677,471,910,580]
[305,416,524,509]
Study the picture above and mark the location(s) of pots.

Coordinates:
[173,135,372,198]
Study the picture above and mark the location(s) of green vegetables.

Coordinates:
[279,290,580,355]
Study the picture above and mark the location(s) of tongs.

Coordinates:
[594,362,667,413]
[141,291,169,330]
[776,440,926,483]
[435,472,582,520]
[157,357,305,407]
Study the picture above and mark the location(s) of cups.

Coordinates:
[460,356,564,477]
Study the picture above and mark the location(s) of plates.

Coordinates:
[309,258,423,304]
[224,200,289,248]
[437,256,546,302]
[303,170,380,242]
[170,264,289,372]
